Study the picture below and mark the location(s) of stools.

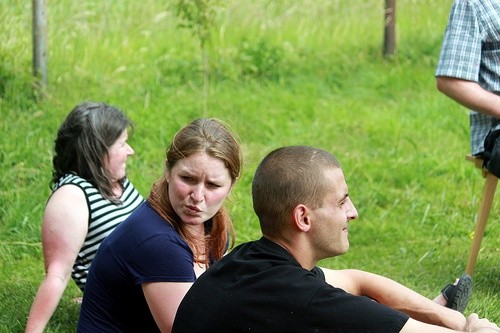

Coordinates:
[465,153,499,275]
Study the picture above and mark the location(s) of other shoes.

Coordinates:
[441,275,472,314]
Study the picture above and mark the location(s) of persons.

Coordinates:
[23,102,472,333]
[171,146,500,333]
[435,0,500,180]
